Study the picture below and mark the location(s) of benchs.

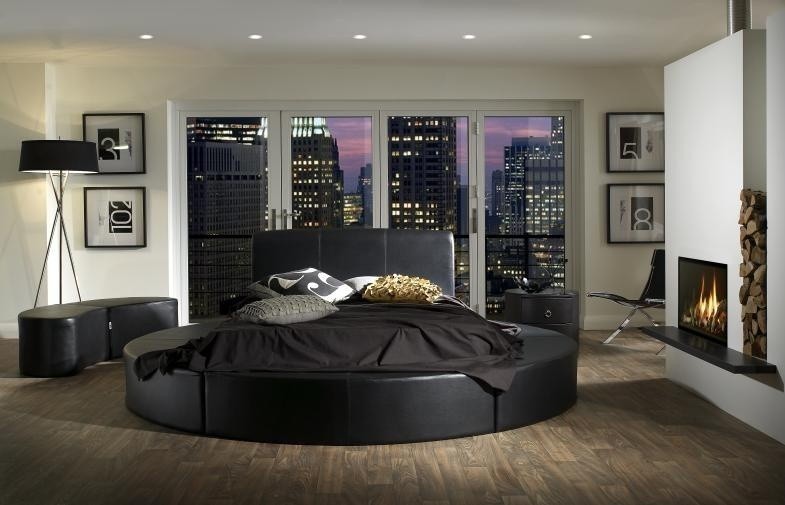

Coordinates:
[17,292,178,377]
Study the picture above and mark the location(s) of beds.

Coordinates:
[123,228,578,446]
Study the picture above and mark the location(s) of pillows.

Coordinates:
[357,273,443,306]
[245,278,283,299]
[230,293,343,325]
[259,266,357,303]
[340,275,381,296]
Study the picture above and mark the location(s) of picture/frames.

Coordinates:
[606,111,665,174]
[608,183,664,243]
[81,112,147,176]
[84,187,145,248]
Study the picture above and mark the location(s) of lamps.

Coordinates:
[18,135,99,307]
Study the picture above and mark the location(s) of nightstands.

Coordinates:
[503,285,580,342]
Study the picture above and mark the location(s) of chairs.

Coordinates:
[586,247,665,345]
